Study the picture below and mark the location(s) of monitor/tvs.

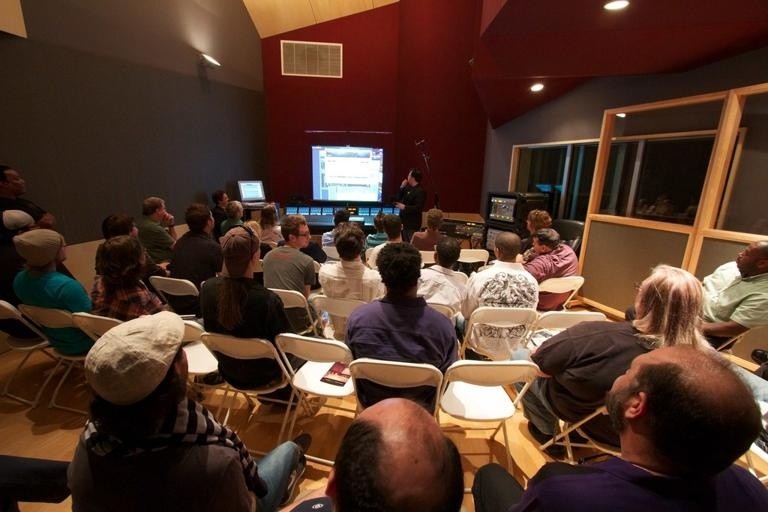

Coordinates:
[285,206,401,217]
[489,196,515,222]
[237,180,267,201]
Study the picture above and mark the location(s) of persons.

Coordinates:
[0,162,767,512]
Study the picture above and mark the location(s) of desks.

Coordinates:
[420,211,486,239]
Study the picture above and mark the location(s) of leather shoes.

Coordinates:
[203,373,225,385]
[751,350,767,366]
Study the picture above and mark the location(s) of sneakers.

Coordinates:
[281,455,312,505]
[293,432,311,460]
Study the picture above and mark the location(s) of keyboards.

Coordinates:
[243,202,268,207]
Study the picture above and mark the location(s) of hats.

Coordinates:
[83,310,186,405]
[11,228,62,267]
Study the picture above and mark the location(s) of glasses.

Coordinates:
[634,281,643,292]
[226,225,253,250]
[298,230,310,237]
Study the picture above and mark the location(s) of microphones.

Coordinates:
[416,139,425,145]
[402,177,411,194]
